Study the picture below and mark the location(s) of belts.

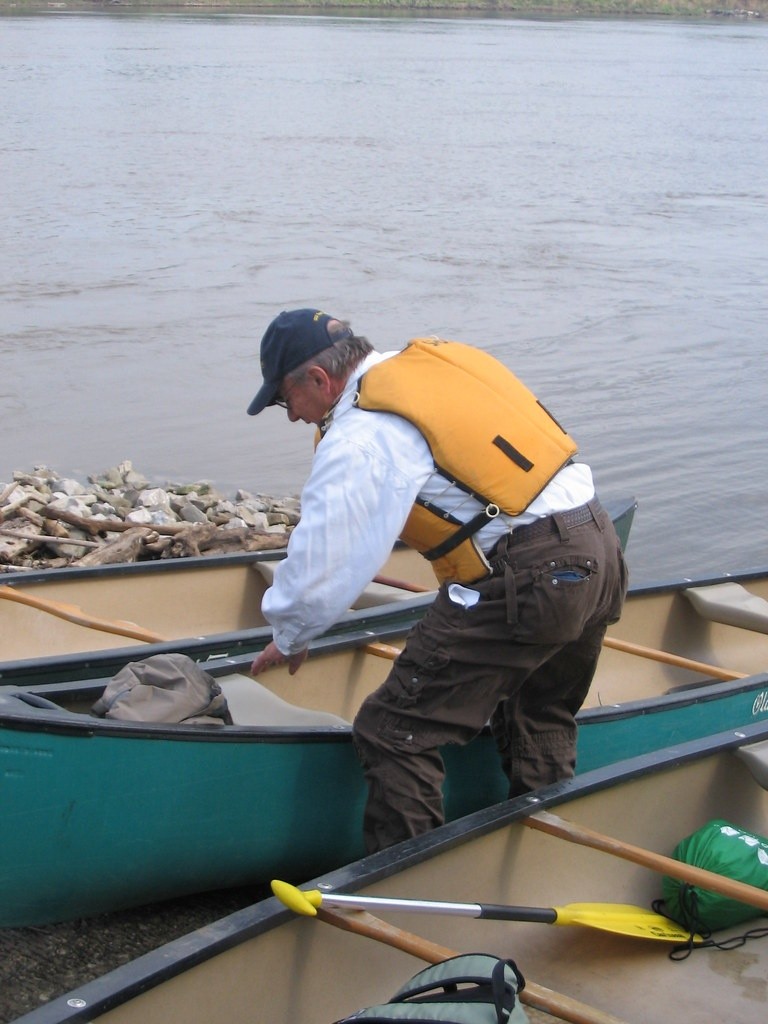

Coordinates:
[485,495,604,560]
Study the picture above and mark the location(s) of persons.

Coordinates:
[246,308,629,854]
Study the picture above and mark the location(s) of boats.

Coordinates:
[0,719,768,1024]
[0,469,639,684]
[0,567,767,932]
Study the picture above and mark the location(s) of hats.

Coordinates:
[246,308,353,416]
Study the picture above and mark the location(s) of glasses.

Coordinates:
[273,381,295,410]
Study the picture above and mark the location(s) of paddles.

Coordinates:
[270,877,703,951]
